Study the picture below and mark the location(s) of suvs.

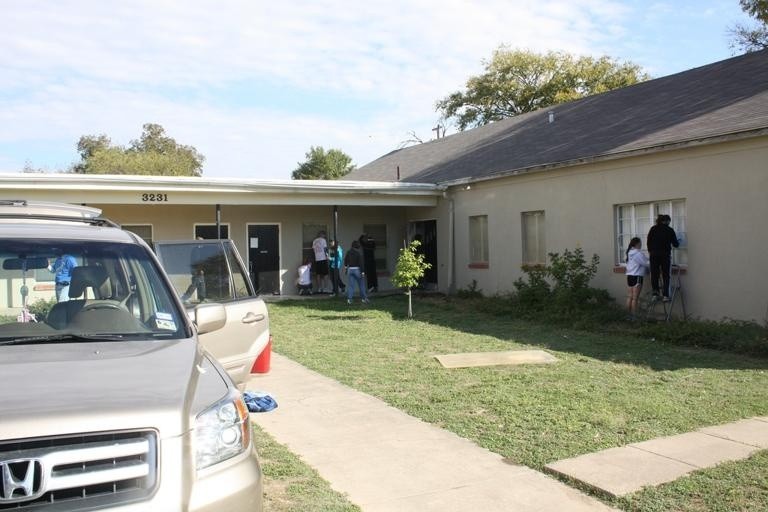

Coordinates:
[0,203,275,511]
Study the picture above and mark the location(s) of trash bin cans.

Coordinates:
[250,335,272,373]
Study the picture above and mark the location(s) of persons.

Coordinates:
[344,240,370,305]
[312,230,331,294]
[179,236,210,304]
[625,237,650,322]
[411,233,428,297]
[47,253,78,303]
[326,239,346,297]
[294,258,313,295]
[646,215,682,302]
[360,232,379,293]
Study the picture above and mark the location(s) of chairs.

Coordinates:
[47,265,133,334]
